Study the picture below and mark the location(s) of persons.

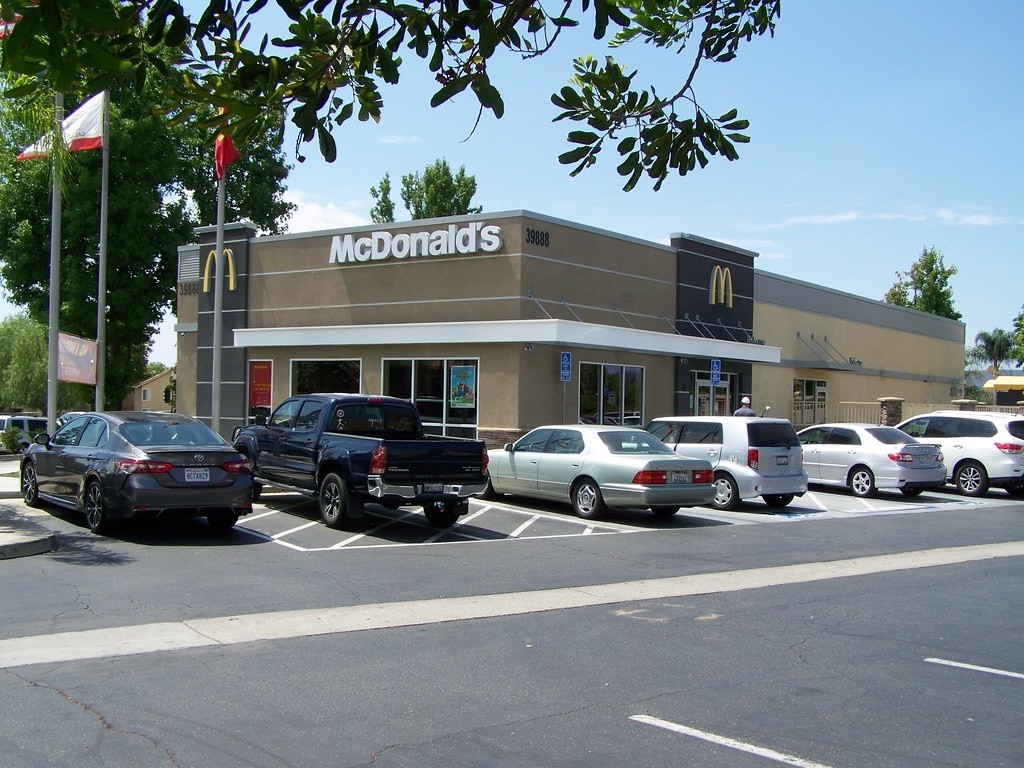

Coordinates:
[733,397,755,416]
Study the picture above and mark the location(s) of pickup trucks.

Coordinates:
[230,392,490,526]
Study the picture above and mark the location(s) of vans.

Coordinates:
[642,417,809,510]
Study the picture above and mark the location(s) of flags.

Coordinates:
[0,0,40,40]
[215,106,238,180]
[16,93,105,161]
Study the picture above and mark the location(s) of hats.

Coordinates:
[741,397,750,404]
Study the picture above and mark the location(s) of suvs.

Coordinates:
[0,414,61,454]
[893,410,1024,497]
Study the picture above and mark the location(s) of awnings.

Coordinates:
[982,375,1024,392]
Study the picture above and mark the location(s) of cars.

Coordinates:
[579,411,642,426]
[16,411,254,530]
[470,425,718,520]
[55,412,88,428]
[794,422,948,498]
